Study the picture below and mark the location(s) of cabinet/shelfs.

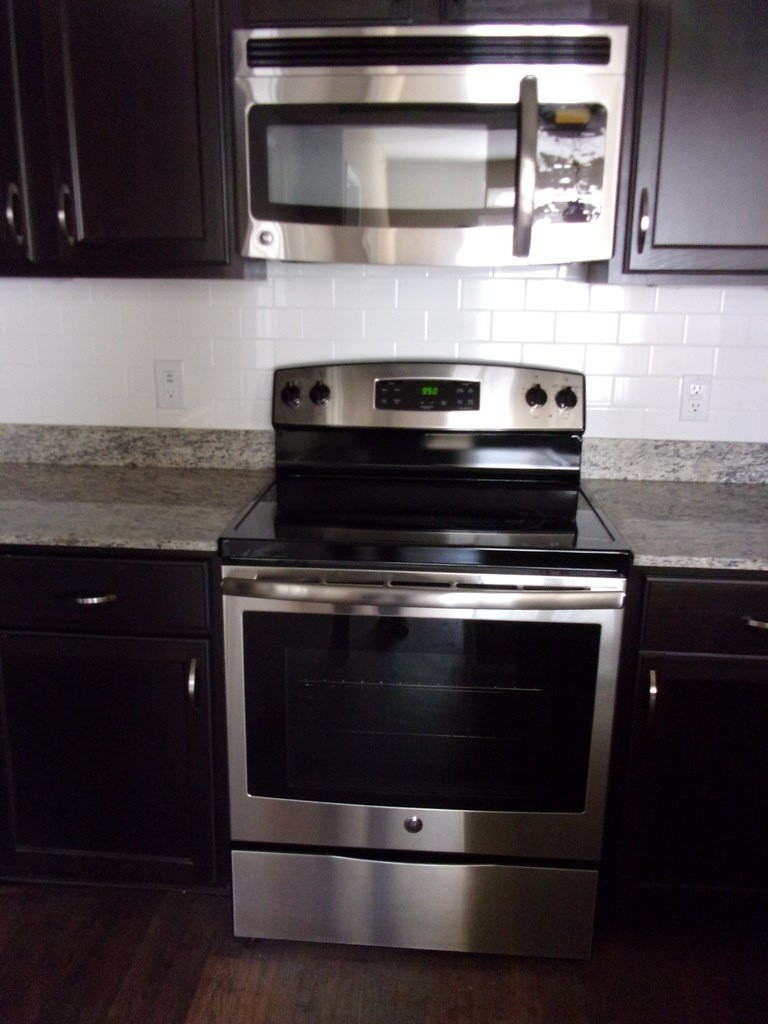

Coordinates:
[0,0,267,280]
[580,435,768,915]
[587,0,768,285]
[0,422,277,896]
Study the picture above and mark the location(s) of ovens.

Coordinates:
[222,562,634,961]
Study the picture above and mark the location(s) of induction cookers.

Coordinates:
[225,362,627,554]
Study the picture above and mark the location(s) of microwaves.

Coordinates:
[233,23,632,270]
